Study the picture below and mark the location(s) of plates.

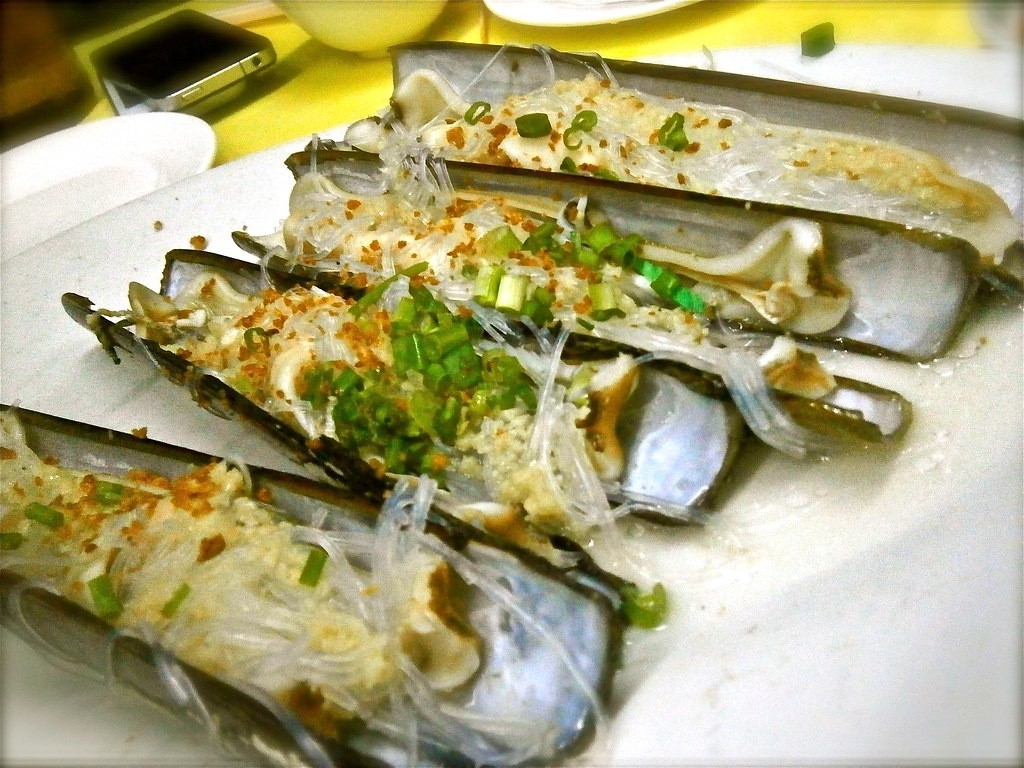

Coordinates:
[482,1,704,27]
[0,39,1024,767]
[1,112,217,258]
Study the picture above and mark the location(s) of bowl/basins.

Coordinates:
[275,0,448,60]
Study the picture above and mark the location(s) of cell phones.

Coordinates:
[91,8,278,112]
